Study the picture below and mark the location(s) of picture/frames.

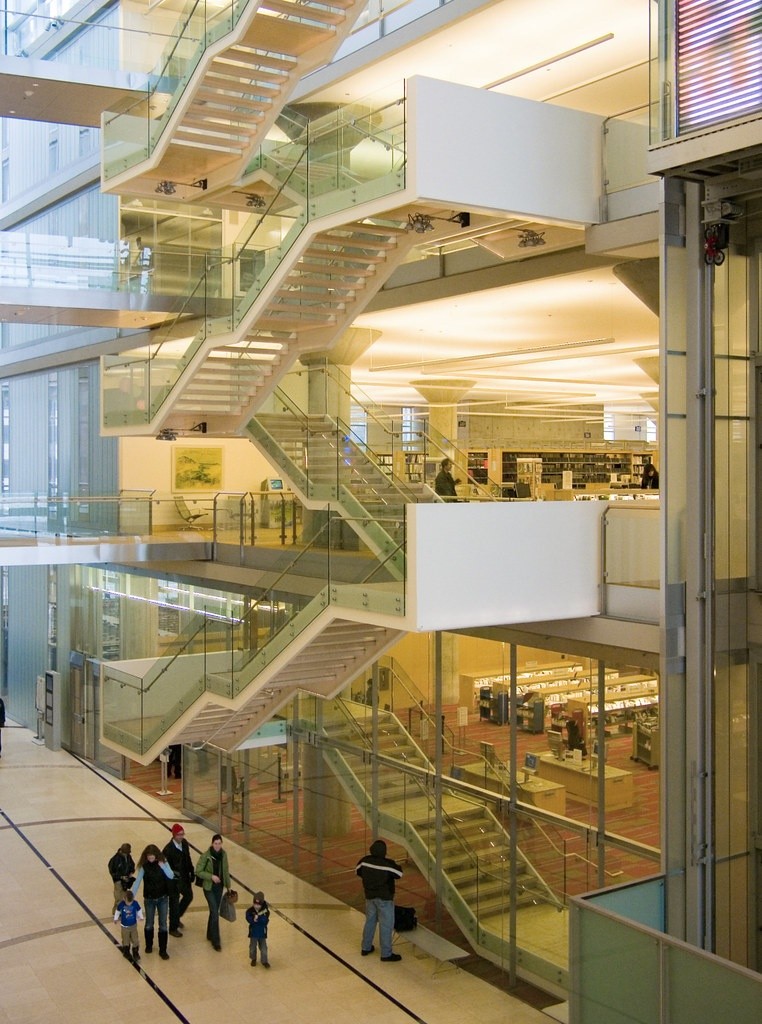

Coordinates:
[170,445,224,493]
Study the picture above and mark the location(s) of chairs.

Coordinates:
[227,495,258,529]
[174,496,208,532]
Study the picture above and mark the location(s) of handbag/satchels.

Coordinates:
[177,866,194,891]
[220,887,238,922]
[195,874,204,887]
[394,905,417,933]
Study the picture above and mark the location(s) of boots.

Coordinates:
[158,930,169,960]
[124,945,140,960]
[144,927,154,953]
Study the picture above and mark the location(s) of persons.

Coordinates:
[113,890,144,962]
[166,744,182,779]
[562,720,588,761]
[161,823,195,938]
[194,833,233,953]
[433,457,462,503]
[244,891,271,969]
[127,843,175,961]
[355,839,404,962]
[366,678,380,709]
[107,843,136,919]
[0,696,7,759]
[641,463,659,495]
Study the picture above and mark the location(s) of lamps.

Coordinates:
[155,180,176,195]
[245,193,266,207]
[15,47,29,57]
[518,228,545,247]
[43,16,63,31]
[156,428,178,441]
[405,210,470,233]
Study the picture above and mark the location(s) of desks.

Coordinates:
[203,508,229,531]
[461,762,567,827]
[525,750,633,810]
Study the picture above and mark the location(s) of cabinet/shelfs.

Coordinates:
[458,661,659,770]
[461,449,654,488]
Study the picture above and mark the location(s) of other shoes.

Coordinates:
[207,927,221,950]
[362,945,374,955]
[381,954,401,961]
[263,963,270,968]
[169,921,184,937]
[251,960,256,966]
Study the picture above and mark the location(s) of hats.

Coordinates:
[172,824,184,834]
[256,891,264,903]
